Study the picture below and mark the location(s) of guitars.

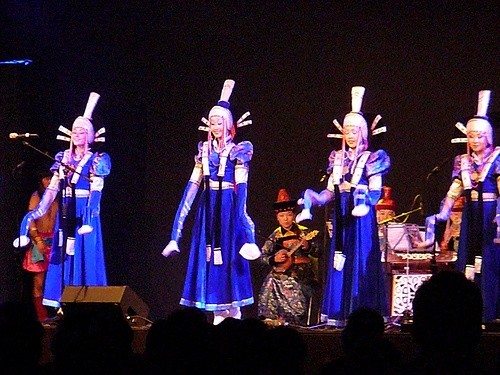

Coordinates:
[271,229,319,272]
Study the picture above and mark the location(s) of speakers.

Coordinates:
[59,285,149,326]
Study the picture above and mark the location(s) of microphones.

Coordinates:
[9,133,40,140]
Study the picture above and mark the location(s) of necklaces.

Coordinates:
[341,150,369,172]
[205,141,231,169]
[468,147,497,168]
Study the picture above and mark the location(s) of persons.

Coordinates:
[376,195,398,262]
[256,205,320,328]
[439,195,464,256]
[26,115,109,325]
[0,269,500,375]
[303,110,394,330]
[435,118,499,334]
[22,169,61,321]
[159,103,257,324]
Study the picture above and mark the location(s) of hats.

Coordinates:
[342,110,369,138]
[374,184,399,211]
[464,115,493,146]
[70,115,95,145]
[273,188,298,214]
[205,101,232,133]
